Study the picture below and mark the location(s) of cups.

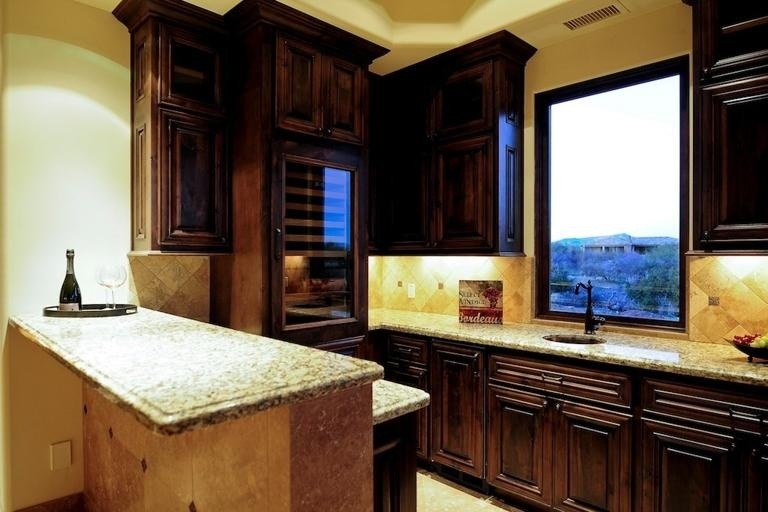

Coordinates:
[99,264,127,309]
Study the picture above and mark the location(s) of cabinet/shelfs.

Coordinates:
[431,339,488,495]
[384,329,430,470]
[638,369,768,512]
[6,303,430,512]
[110,1,231,255]
[208,0,391,362]
[683,0,768,255]
[487,352,634,511]
[368,29,539,257]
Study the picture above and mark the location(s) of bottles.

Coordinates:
[60,249,81,311]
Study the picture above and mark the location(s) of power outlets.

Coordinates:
[51,440,75,473]
[407,283,418,298]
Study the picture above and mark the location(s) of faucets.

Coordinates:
[574,279,605,334]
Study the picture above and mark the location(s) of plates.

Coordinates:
[722,337,768,362]
[44,304,137,317]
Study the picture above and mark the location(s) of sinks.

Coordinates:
[541,333,606,345]
